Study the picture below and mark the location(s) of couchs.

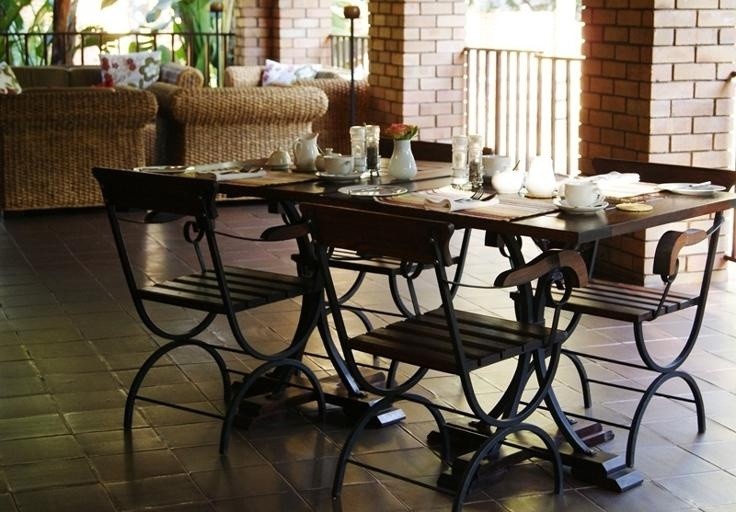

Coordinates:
[172,85,329,170]
[225,65,371,156]
[147,59,204,112]
[1,86,157,211]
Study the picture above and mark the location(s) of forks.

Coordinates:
[220,164,252,175]
[471,190,485,200]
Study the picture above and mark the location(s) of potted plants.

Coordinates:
[386,123,418,180]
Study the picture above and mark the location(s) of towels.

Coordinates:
[423,193,500,214]
[581,171,640,190]
[194,169,267,183]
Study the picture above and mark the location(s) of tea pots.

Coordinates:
[293,132,320,171]
[522,157,581,200]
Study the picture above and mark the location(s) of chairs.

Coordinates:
[294,201,568,512]
[506,158,736,469]
[90,165,326,457]
[293,139,494,389]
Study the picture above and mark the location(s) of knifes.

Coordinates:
[481,192,497,201]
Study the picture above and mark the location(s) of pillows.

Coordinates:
[261,58,322,87]
[98,50,161,90]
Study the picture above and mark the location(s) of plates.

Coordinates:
[555,200,609,214]
[317,172,361,183]
[133,165,190,174]
[659,182,726,195]
[616,203,653,211]
[265,163,290,170]
[338,184,409,196]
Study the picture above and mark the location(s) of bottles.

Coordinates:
[366,125,380,170]
[451,135,468,185]
[350,126,366,173]
[468,134,483,186]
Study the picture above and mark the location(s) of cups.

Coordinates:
[324,155,355,175]
[565,183,602,207]
[483,155,511,176]
